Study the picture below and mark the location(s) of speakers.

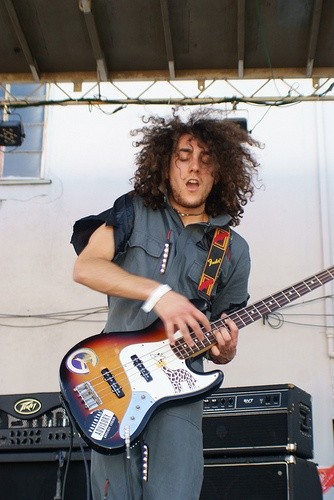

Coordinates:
[197,455,324,500]
[0,450,95,500]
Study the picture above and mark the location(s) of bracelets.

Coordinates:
[141,284,172,313]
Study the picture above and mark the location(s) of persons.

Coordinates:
[69,105,267,500]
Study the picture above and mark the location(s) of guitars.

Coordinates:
[59,267,334,456]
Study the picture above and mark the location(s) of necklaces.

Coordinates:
[179,210,206,217]
